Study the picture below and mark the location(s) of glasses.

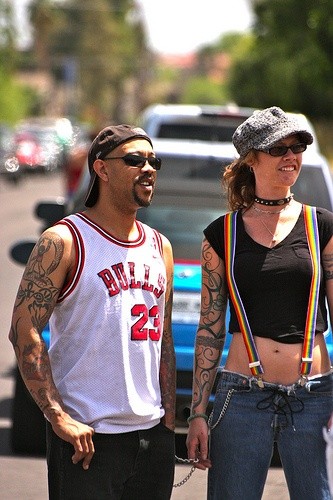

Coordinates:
[253,141,307,157]
[101,154,162,170]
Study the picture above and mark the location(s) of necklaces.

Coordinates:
[253,205,277,241]
[254,193,293,206]
[253,204,287,214]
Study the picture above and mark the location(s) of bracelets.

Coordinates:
[187,414,208,424]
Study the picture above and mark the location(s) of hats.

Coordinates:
[83,124,153,208]
[232,106,314,157]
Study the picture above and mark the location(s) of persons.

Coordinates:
[8,125,176,500]
[187,107,333,500]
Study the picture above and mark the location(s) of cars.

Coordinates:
[10,102,333,468]
